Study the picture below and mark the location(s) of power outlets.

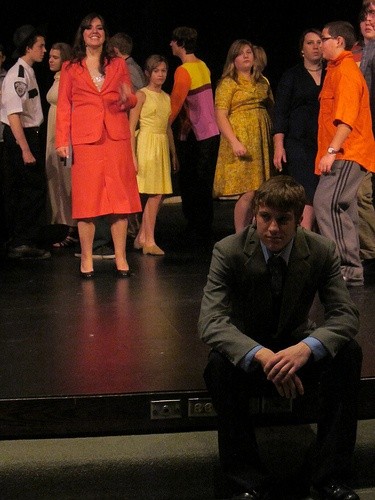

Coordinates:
[149,396,295,420]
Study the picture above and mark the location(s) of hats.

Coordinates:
[12,25,34,58]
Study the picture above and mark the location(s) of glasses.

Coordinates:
[321,36,337,43]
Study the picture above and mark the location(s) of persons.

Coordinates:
[313,22,375,287]
[75,33,146,259]
[168,27,221,252]
[197,176,365,500]
[0,27,51,261]
[129,54,179,255]
[46,43,80,246]
[351,0,375,209]
[273,27,329,233]
[54,13,142,278]
[213,41,274,234]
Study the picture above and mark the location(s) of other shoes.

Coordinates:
[363,260,375,276]
[8,245,51,260]
[219,194,241,200]
[112,262,133,277]
[133,238,165,256]
[162,191,182,204]
[80,268,96,279]
[346,279,363,286]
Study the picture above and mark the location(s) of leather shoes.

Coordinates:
[302,470,360,500]
[232,479,276,500]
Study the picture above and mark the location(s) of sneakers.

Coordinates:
[73,243,116,259]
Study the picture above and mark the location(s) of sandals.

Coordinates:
[53,231,81,247]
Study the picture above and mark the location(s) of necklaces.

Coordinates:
[306,65,322,72]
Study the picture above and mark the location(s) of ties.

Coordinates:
[267,255,286,277]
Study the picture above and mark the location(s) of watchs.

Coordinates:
[327,146,339,154]
